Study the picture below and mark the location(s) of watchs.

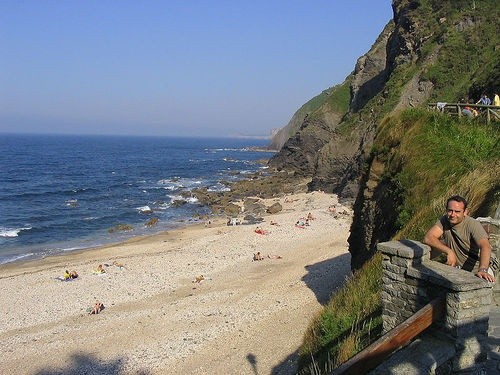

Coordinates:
[478,268,488,273]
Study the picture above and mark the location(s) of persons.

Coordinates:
[465,101,477,117]
[307,213,316,221]
[253,252,265,261]
[194,274,204,283]
[70,271,78,279]
[326,206,336,212]
[270,221,280,226]
[295,221,306,229]
[256,226,272,235]
[57,270,70,281]
[110,261,129,271]
[423,196,496,282]
[492,91,500,111]
[98,264,106,274]
[266,254,281,260]
[476,93,491,112]
[90,303,101,314]
